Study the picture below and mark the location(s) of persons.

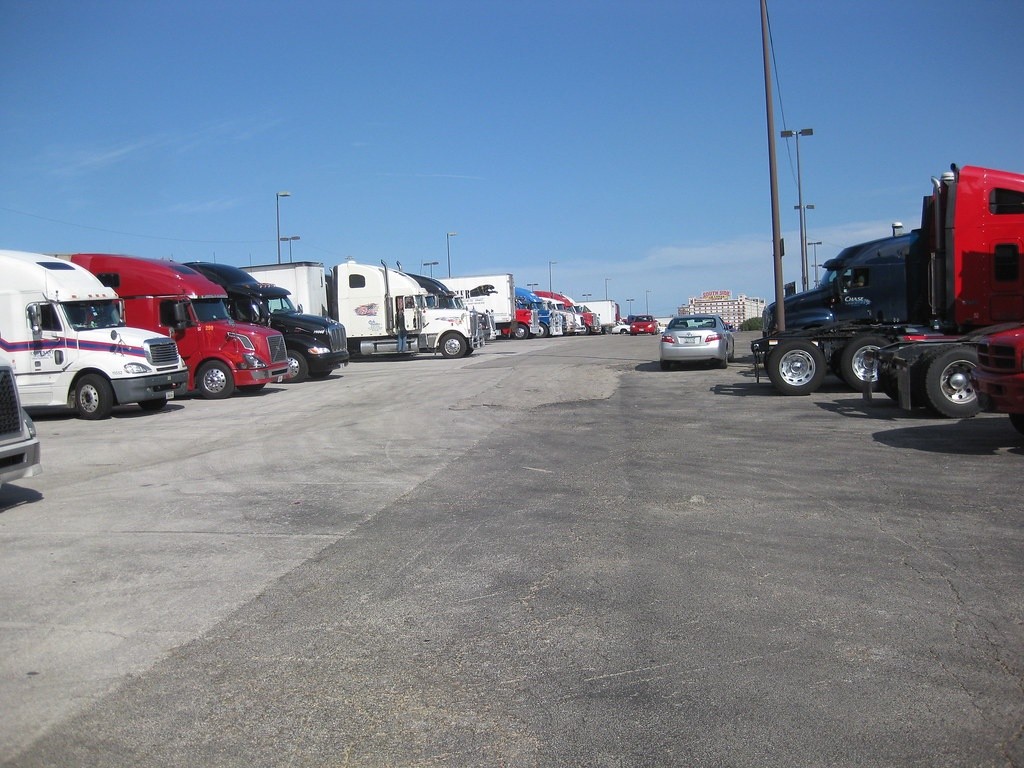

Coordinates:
[395,307,406,353]
[852,272,865,287]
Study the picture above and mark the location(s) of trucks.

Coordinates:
[180,261,347,386]
[0,248,191,422]
[40,252,293,400]
[236,260,634,361]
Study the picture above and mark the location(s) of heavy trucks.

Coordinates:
[748,162,1024,444]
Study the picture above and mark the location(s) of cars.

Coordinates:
[611,322,630,335]
[629,315,659,336]
[659,323,670,333]
[0,352,44,492]
[658,314,735,369]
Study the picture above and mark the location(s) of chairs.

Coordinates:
[702,323,712,327]
[680,322,688,327]
[675,324,685,330]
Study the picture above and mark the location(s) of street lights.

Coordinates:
[422,262,439,278]
[582,293,592,304]
[605,277,613,300]
[447,232,458,279]
[646,289,653,315]
[276,191,291,265]
[527,282,538,293]
[281,236,302,263]
[891,220,903,237]
[548,260,557,294]
[793,204,817,293]
[806,240,822,287]
[625,299,636,315]
[780,129,814,289]
